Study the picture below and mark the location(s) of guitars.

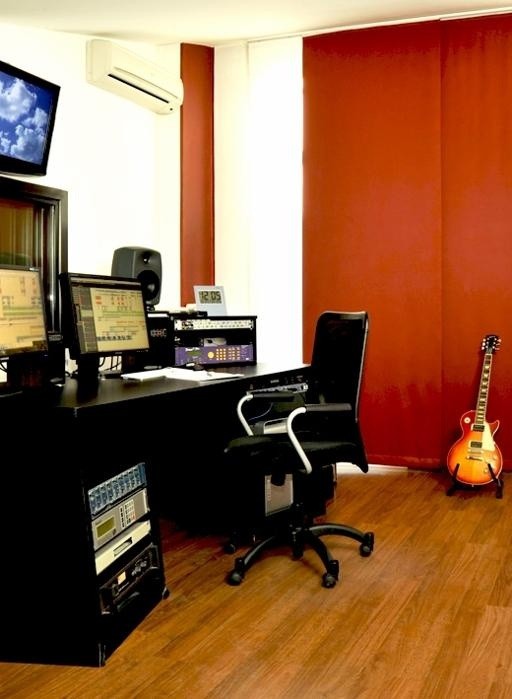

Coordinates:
[447,335,504,487]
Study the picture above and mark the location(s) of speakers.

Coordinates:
[112,247,162,305]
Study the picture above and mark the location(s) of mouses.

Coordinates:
[193,365,204,371]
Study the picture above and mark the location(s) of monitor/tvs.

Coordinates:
[0,262,51,384]
[0,60,61,177]
[58,271,151,378]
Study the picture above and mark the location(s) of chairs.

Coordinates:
[214,308,372,591]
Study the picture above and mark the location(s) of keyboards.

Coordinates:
[121,367,192,380]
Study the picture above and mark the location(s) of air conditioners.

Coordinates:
[87,42,186,120]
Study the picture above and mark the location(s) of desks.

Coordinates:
[1,358,308,669]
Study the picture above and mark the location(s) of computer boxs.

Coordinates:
[250,418,295,517]
